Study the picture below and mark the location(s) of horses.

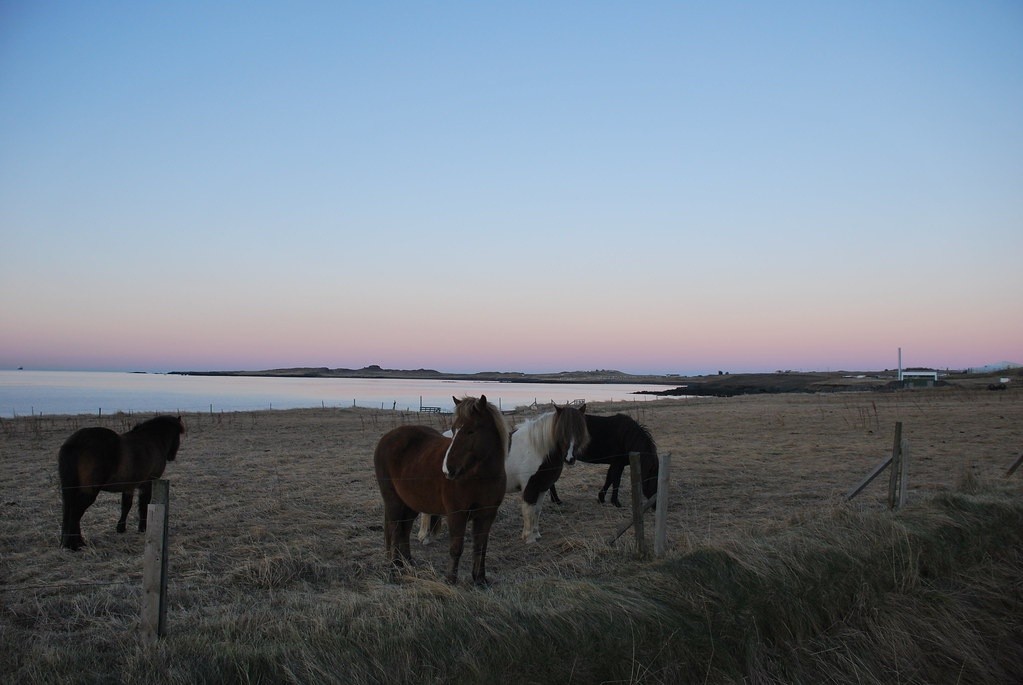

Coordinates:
[374,395,659,589]
[58,414,186,549]
